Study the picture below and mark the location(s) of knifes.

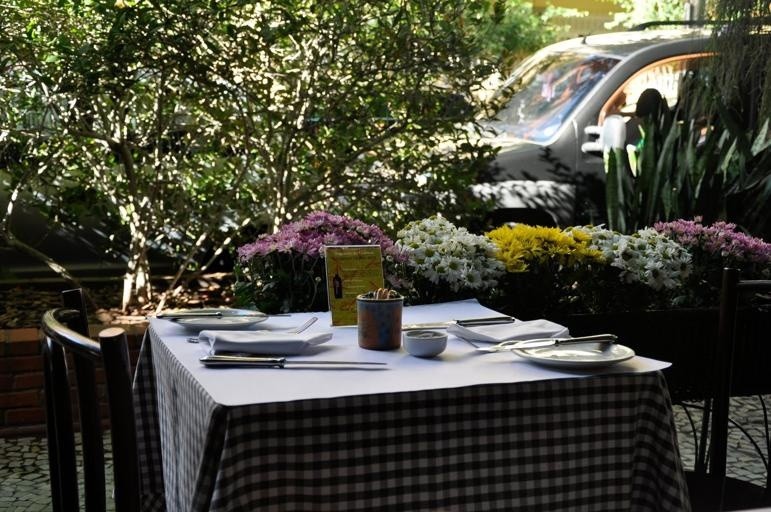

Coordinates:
[198,354,389,369]
[155,312,291,320]
[476,332,618,352]
[399,316,516,331]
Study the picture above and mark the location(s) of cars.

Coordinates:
[439,22,771,227]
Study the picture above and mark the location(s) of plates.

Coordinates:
[511,337,637,371]
[169,307,267,331]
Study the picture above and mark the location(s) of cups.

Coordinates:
[355,295,403,351]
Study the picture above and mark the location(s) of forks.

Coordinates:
[185,316,318,344]
[455,334,523,355]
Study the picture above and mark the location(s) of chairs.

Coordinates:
[37,288,145,512]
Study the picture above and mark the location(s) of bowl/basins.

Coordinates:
[403,329,448,358]
